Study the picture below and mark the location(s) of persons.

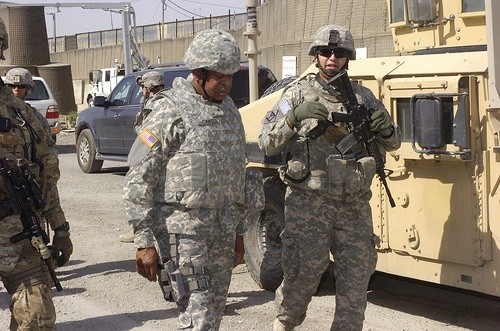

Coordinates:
[0,16,73,331]
[5,68,57,154]
[259,25,402,331]
[122,29,246,331]
[119,72,165,241]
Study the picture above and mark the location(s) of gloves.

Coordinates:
[52,234,73,267]
[367,108,392,136]
[287,96,329,126]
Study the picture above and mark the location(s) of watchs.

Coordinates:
[55,221,69,231]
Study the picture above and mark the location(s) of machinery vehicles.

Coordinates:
[242,47,497,296]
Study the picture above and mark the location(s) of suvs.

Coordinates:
[1,77,62,143]
[75,60,278,174]
[86,64,144,107]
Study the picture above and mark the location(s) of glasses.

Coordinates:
[317,48,350,58]
[7,84,29,89]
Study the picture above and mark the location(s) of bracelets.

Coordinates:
[380,124,395,139]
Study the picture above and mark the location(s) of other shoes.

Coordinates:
[273,318,294,331]
[119,233,135,243]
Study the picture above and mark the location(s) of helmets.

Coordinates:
[183,29,240,74]
[136,71,165,88]
[0,17,9,50]
[308,25,356,60]
[4,67,34,90]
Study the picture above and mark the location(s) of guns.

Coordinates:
[0,155,63,293]
[325,71,397,208]
